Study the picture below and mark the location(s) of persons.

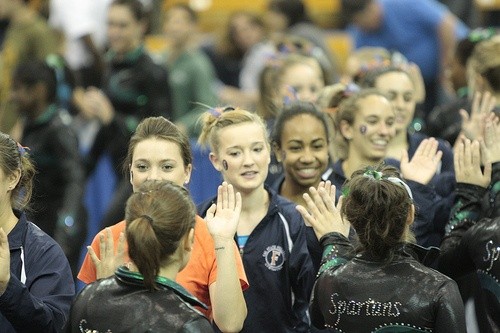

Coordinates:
[0,0,500,333]
